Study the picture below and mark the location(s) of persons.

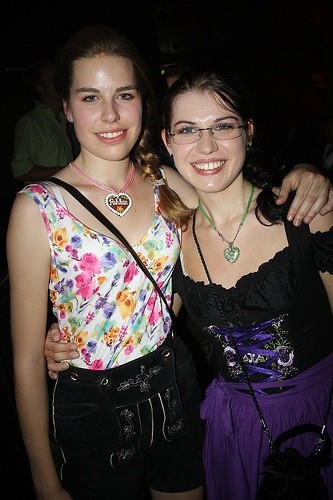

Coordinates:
[11,64,74,188]
[7,25,333,500]
[43,66,333,500]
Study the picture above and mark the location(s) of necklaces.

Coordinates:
[68,162,133,218]
[199,182,254,262]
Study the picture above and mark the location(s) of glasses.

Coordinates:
[165,122,249,144]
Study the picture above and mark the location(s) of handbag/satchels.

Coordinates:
[258,424,333,500]
[171,332,202,439]
